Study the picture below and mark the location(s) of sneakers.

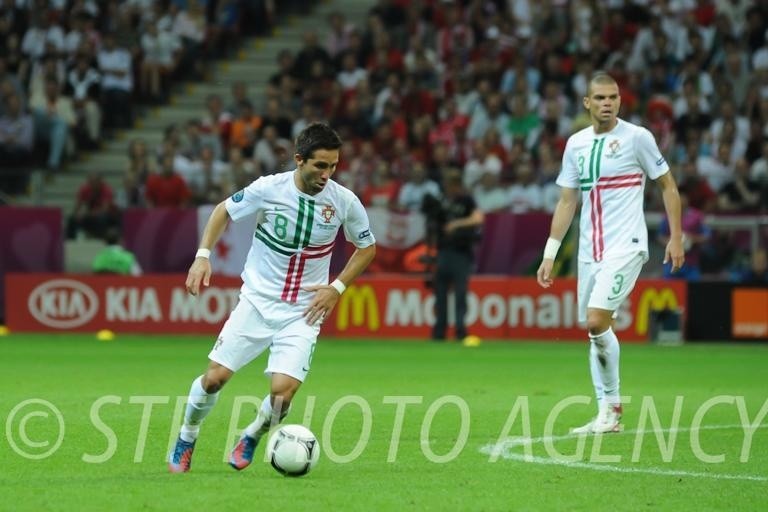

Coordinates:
[228,434,257,469]
[572,406,622,433]
[169,433,195,472]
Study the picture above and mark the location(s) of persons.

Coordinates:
[532,69,688,435]
[168,123,378,476]
[426,166,486,342]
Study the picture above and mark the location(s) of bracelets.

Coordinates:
[330,278,347,295]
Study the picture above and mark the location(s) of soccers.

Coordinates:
[265,423,320,476]
